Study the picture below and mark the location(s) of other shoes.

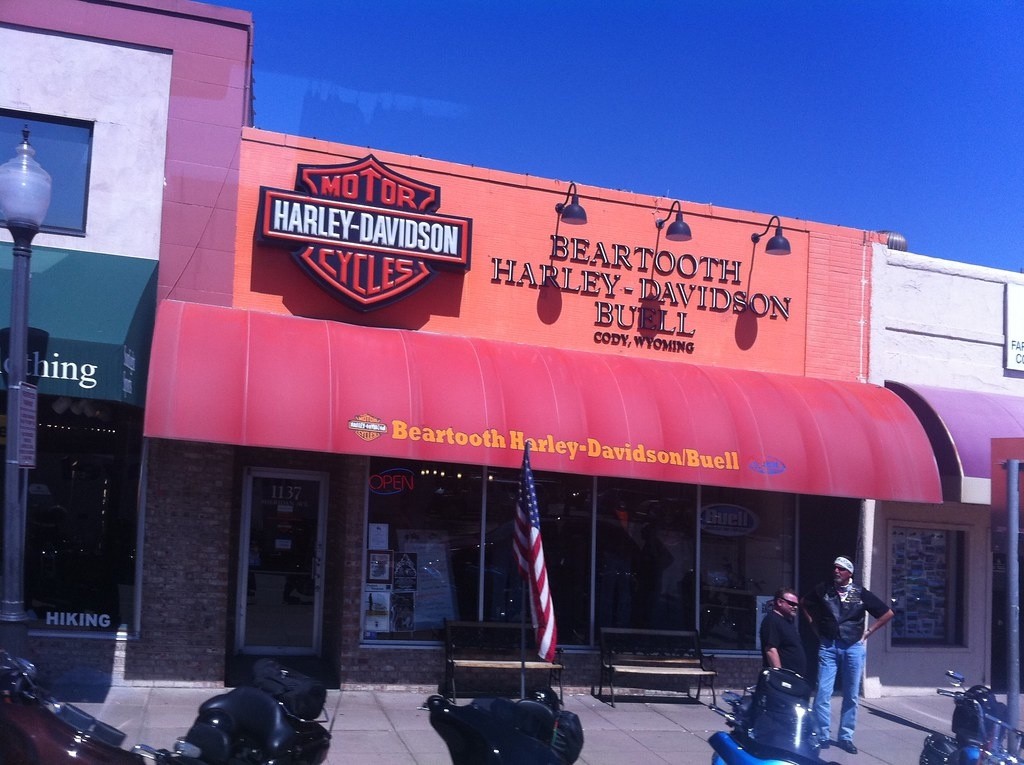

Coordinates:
[840,740,857,754]
[818,739,830,749]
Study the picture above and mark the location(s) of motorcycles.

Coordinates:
[429,689,584,765]
[920,670,1024,765]
[0,646,332,765]
[708,666,839,765]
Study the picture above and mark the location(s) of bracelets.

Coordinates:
[867,629,872,633]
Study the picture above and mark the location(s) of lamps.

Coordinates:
[655,201,691,241]
[750,215,790,255]
[554,182,587,225]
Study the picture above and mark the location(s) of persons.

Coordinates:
[759,556,894,754]
[595,494,694,627]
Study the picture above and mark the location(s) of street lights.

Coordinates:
[0,128,51,658]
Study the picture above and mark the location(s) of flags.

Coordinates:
[512,442,557,663]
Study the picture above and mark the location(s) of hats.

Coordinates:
[833,556,854,574]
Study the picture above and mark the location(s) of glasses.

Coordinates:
[779,596,800,607]
[833,566,848,571]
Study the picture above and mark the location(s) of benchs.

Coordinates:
[597,628,717,709]
[442,618,563,706]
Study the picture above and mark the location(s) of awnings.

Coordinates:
[144,300,944,504]
[884,379,1024,507]
